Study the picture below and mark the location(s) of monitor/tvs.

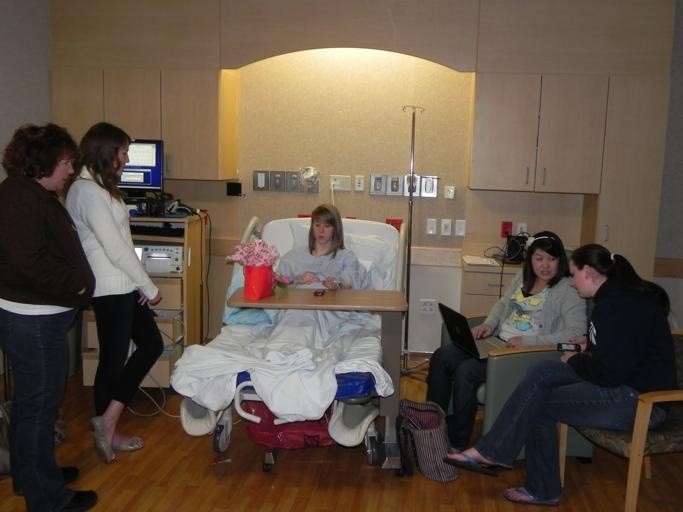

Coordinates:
[109,139,164,200]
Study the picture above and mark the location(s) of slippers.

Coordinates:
[503,486,559,505]
[443,452,497,477]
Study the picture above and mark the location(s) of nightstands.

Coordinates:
[459,255,522,317]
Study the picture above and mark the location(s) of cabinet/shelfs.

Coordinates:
[102,70,239,182]
[462,72,612,194]
[82,214,201,390]
[54,69,103,148]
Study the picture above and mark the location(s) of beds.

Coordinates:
[170,214,412,475]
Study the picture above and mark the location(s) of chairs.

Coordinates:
[553,329,683,512]
[431,248,595,441]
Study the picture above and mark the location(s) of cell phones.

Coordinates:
[558,342,581,352]
[313,288,325,296]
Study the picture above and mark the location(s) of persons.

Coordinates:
[264,203,358,371]
[0,122,98,512]
[443,244,677,506]
[396,231,588,477]
[59,122,163,465]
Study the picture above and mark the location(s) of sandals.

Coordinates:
[91,414,114,463]
[112,436,144,451]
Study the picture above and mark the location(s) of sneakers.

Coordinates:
[60,467,79,486]
[60,490,97,511]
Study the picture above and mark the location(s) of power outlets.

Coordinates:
[420,299,438,315]
[330,175,350,191]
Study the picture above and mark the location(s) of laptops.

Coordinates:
[438,302,515,360]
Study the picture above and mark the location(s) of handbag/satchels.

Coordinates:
[398,398,458,482]
